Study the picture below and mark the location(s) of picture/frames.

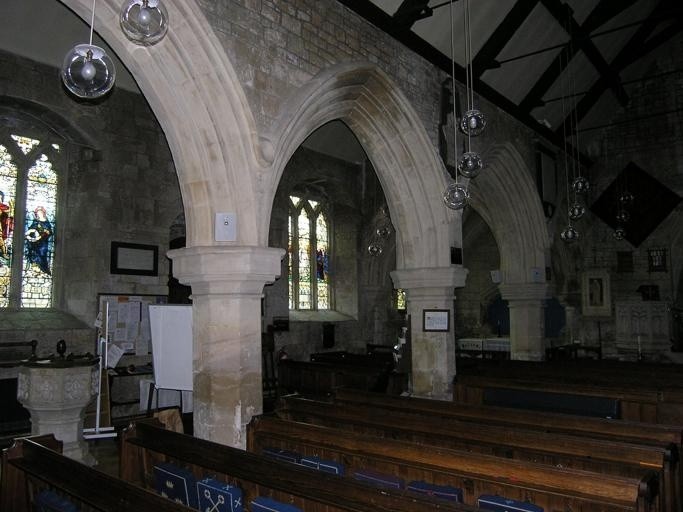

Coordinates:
[110,241,158,276]
[581,268,612,317]
[423,309,450,332]
[640,285,660,301]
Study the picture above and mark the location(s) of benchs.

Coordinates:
[295,361,369,394]
[118,418,494,512]
[247,374,683,511]
[1,433,200,512]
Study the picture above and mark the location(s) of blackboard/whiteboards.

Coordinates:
[148,304,193,392]
[96,292,167,356]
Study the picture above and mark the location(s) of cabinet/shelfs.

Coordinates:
[84,370,111,426]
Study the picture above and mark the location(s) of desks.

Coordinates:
[457,336,570,368]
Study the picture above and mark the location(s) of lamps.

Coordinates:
[612,190,635,241]
[442,0,486,210]
[560,13,589,244]
[61,0,169,99]
[368,173,396,256]
